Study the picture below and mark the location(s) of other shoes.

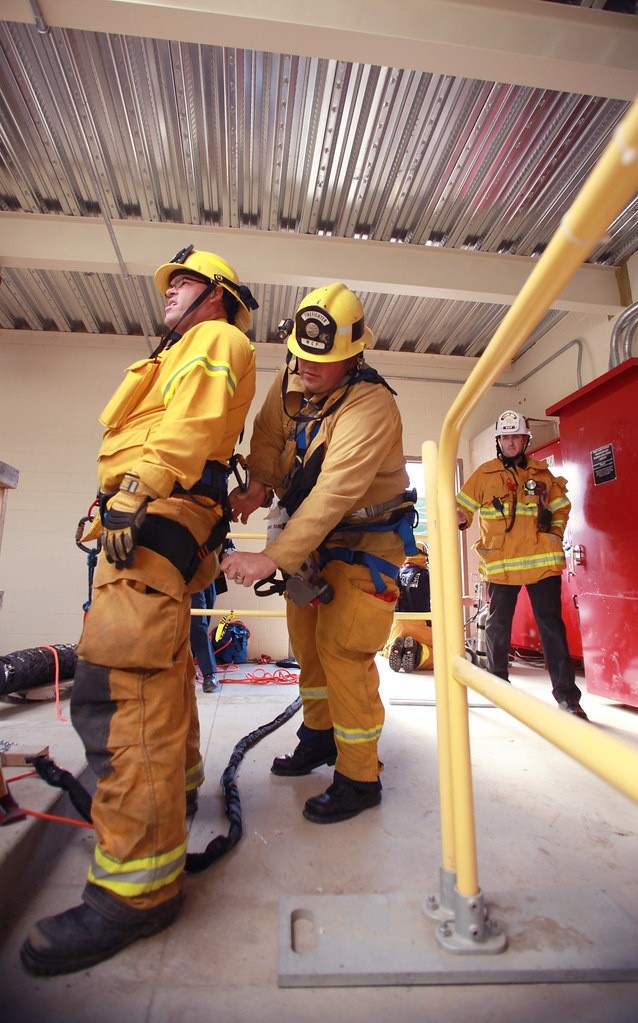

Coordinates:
[203,672,219,692]
[184,787,199,816]
[559,699,587,719]
[388,637,405,672]
[402,636,417,673]
[20,894,183,975]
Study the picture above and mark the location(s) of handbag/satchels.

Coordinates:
[264,500,316,586]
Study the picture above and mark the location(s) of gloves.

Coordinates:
[100,475,159,570]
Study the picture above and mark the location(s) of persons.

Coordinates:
[395,542,431,612]
[19,244,258,974]
[190,521,235,693]
[219,282,417,825]
[456,410,590,723]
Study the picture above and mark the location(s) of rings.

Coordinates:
[237,576,242,580]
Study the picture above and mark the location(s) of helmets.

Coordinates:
[415,540,429,562]
[494,411,531,438]
[154,244,259,334]
[277,282,374,363]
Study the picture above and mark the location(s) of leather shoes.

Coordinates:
[271,740,337,776]
[302,778,381,823]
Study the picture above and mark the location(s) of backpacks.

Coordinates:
[211,615,251,664]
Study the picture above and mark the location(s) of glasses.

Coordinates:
[163,277,210,299]
